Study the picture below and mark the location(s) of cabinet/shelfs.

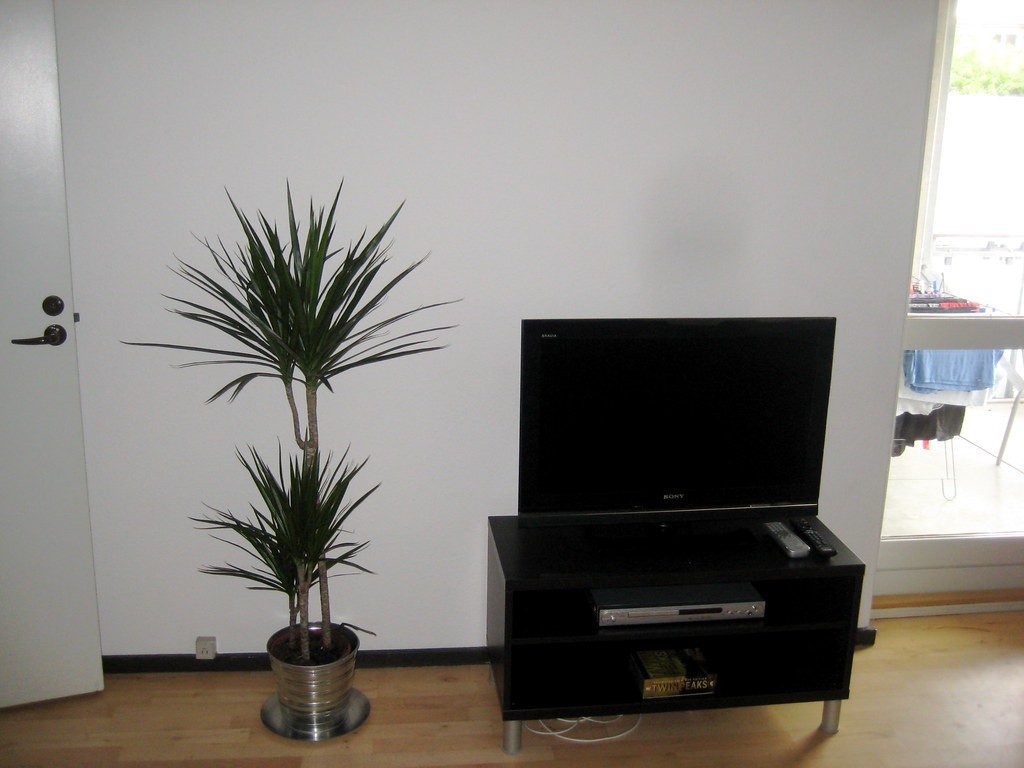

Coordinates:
[487,517,866,754]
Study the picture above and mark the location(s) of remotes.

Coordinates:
[761,521,810,558]
[789,518,836,557]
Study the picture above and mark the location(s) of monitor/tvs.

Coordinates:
[518,317,840,553]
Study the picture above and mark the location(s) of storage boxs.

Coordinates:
[630,647,718,700]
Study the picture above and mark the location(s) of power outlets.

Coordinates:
[196,637,216,660]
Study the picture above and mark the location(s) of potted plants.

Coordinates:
[120,176,466,747]
